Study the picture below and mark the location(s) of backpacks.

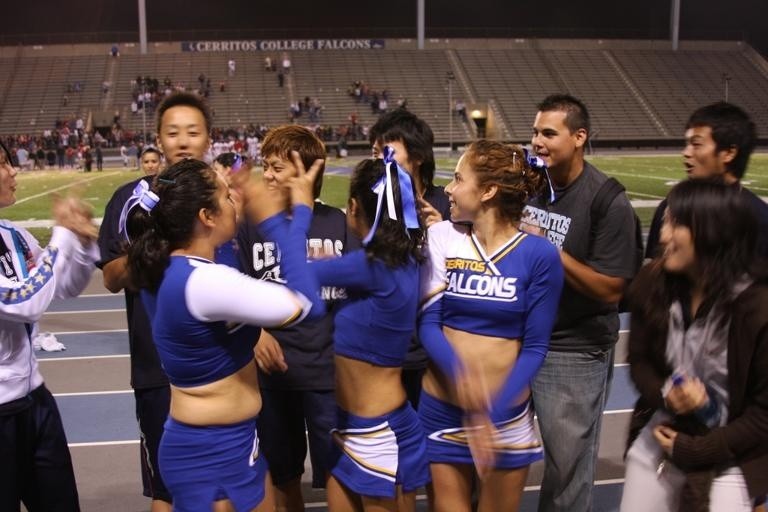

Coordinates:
[587,175,645,311]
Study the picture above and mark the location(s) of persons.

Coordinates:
[0,143,103,512]
[108,44,120,58]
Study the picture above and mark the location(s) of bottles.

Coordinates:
[670,366,719,426]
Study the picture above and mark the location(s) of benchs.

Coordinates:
[0,46,766,159]
